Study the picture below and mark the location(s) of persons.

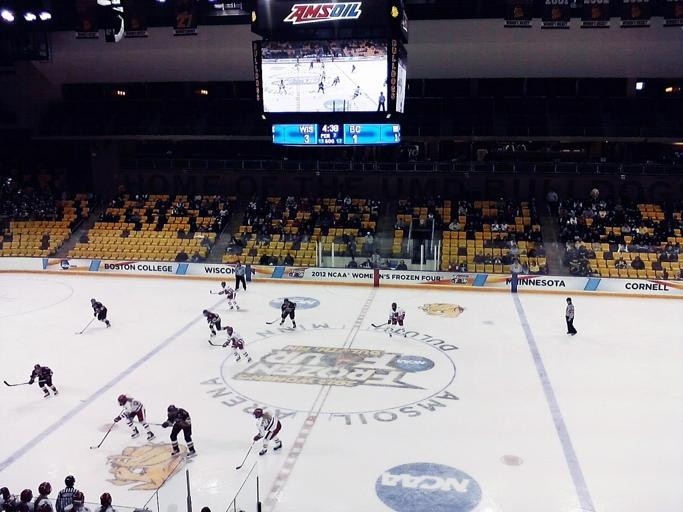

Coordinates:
[278,298,297,328]
[189,250,204,263]
[251,407,282,455]
[160,405,196,455]
[174,249,188,261]
[221,326,250,362]
[27,363,59,397]
[387,302,407,338]
[112,393,154,441]
[217,281,240,310]
[222,191,382,291]
[0,174,222,242]
[200,505,211,512]
[565,297,578,335]
[386,188,681,274]
[258,38,406,115]
[90,298,111,327]
[201,309,229,338]
[0,475,115,512]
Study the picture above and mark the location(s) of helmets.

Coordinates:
[117,395,127,404]
[1,475,113,506]
[167,406,176,415]
[90,299,95,304]
[254,408,262,418]
[34,365,40,372]
[226,327,233,335]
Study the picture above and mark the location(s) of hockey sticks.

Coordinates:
[371,323,387,327]
[4,380,45,386]
[266,317,281,324]
[74,318,94,334]
[236,441,254,469]
[90,410,123,449]
[209,290,219,294]
[208,340,230,346]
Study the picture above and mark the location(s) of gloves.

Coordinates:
[113,416,120,422]
[253,435,259,441]
[161,422,168,428]
[28,379,34,384]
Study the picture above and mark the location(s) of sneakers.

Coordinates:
[43,390,58,398]
[235,356,251,362]
[170,449,195,458]
[130,431,155,441]
[258,444,282,455]
